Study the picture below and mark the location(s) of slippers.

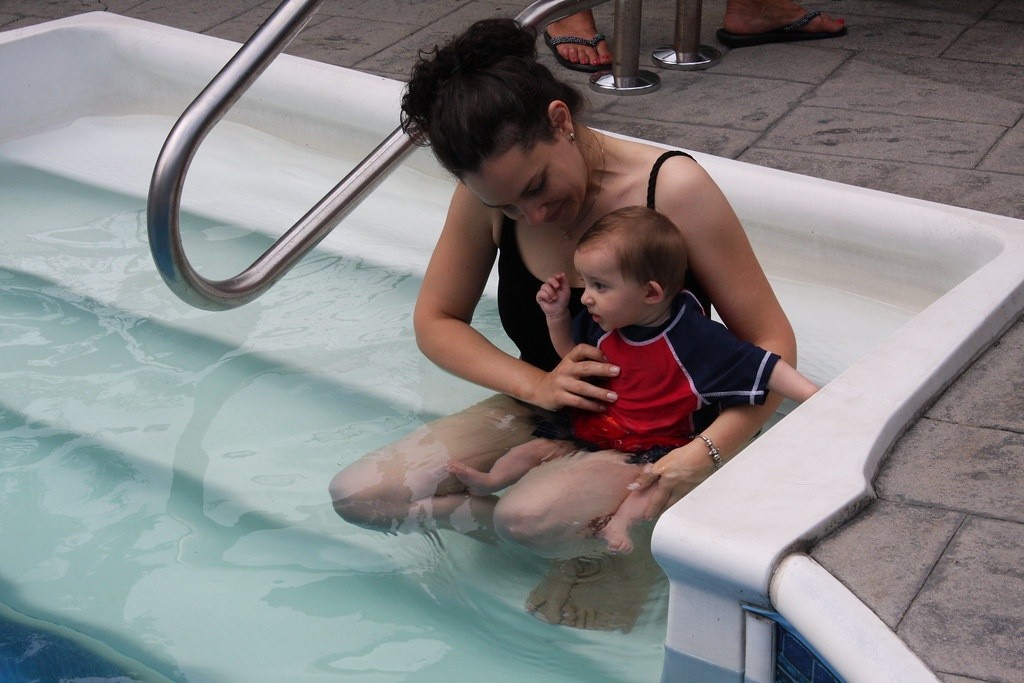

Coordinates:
[543,13,614,73]
[715,1,848,49]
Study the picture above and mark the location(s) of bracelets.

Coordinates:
[694,434,724,470]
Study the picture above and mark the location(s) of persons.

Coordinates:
[444,205,820,557]
[330,18,798,636]
[544,0,848,74]
